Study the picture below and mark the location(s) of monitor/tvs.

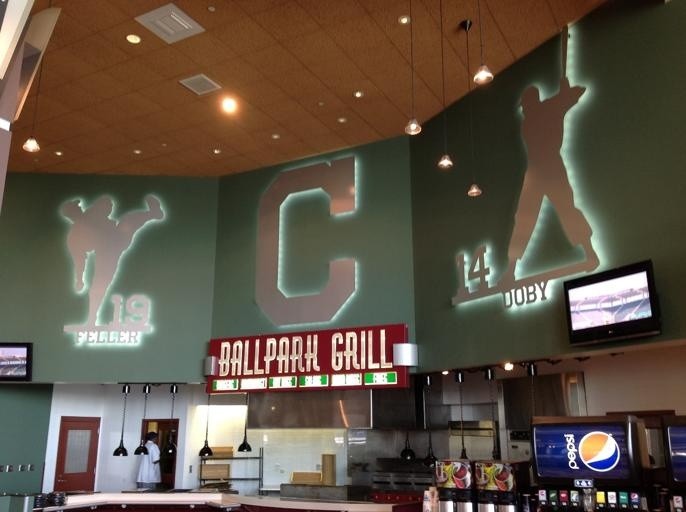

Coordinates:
[564,259,661,348]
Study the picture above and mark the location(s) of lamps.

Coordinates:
[112,383,253,459]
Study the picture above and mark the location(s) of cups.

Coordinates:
[495,468,513,492]
[451,465,470,488]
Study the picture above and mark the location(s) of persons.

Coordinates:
[136,433,162,488]
